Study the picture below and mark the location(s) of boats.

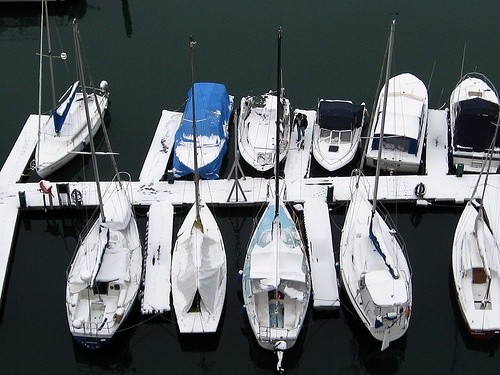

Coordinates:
[449,65,500,175]
[171,81,234,180]
[311,98,366,173]
[238,87,290,174]
[365,73,429,176]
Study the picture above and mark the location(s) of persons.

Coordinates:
[293,113,308,142]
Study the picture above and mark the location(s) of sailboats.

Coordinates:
[452,86,500,342]
[34,0,109,182]
[66,18,145,350]
[243,24,314,375]
[339,19,412,343]
[170,34,227,334]
